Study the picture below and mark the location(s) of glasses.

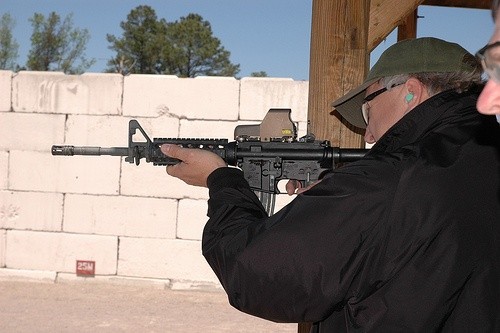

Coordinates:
[361,81,406,126]
[476,42,499,64]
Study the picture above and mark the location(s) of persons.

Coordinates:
[161,36,500,332]
[476,0,500,332]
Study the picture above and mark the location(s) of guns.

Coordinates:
[51,109,371,218]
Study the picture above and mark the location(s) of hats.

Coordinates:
[332,37,483,131]
